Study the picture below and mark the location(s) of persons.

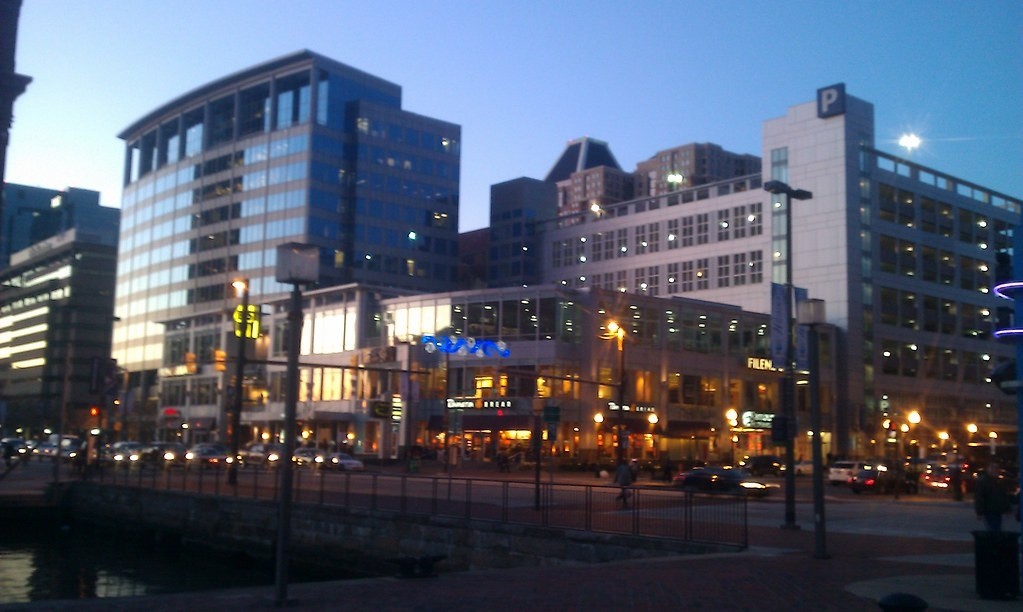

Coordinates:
[630,457,639,482]
[663,457,673,483]
[613,458,634,505]
[974,461,1012,530]
[496,443,521,474]
[4,443,13,467]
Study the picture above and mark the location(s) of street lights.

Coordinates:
[763,179,814,530]
[597,320,625,482]
[226,277,251,486]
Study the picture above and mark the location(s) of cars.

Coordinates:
[737,454,805,478]
[0,432,365,474]
[829,456,975,498]
[671,464,770,498]
